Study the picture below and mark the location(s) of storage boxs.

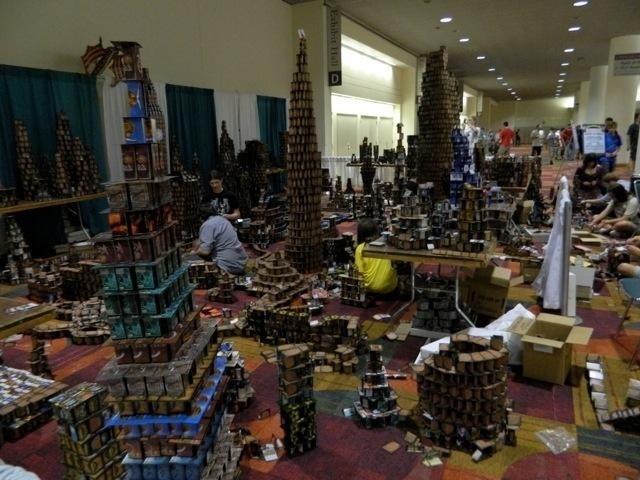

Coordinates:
[509,307,598,393]
[568,258,596,301]
[572,230,601,249]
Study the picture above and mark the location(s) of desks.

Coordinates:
[0,184,129,277]
[354,154,541,350]
[0,297,58,374]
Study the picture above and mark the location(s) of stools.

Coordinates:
[612,269,639,371]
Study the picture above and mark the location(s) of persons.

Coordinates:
[459,108,640,302]
[189,202,248,276]
[202,171,240,228]
